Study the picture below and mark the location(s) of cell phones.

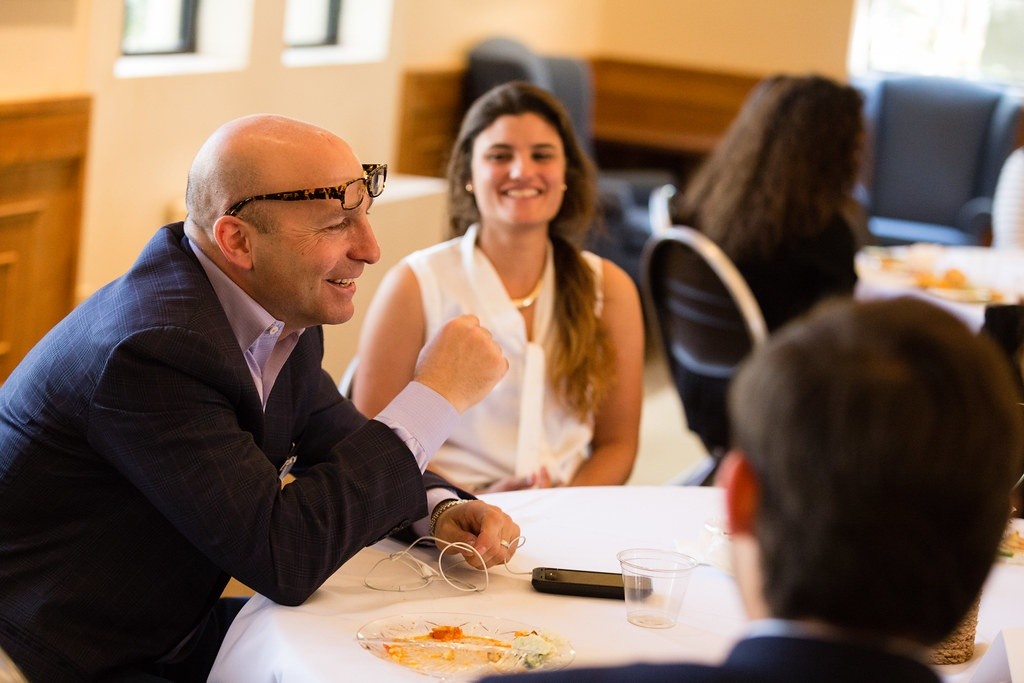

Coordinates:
[531,567,654,601]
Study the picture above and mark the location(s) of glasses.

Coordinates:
[223,163,387,217]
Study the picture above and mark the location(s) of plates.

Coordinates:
[996,529,1024,566]
[868,249,1006,302]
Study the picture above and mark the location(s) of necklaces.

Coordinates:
[510,277,544,309]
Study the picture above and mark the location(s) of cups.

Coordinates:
[615,547,698,630]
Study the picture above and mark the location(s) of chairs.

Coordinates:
[857,74,1021,246]
[637,225,771,486]
[463,36,647,280]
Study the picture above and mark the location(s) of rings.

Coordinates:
[500,538,510,548]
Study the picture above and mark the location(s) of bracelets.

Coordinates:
[475,477,500,495]
[430,499,474,537]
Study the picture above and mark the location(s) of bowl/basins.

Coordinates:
[357,614,575,683]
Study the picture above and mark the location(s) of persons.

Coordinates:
[670,77,868,337]
[354,82,642,497]
[479,295,1024,683]
[0,113,520,683]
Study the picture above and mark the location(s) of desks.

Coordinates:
[847,241,1024,333]
[206,487,1024,683]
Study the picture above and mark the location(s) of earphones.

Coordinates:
[420,565,433,580]
